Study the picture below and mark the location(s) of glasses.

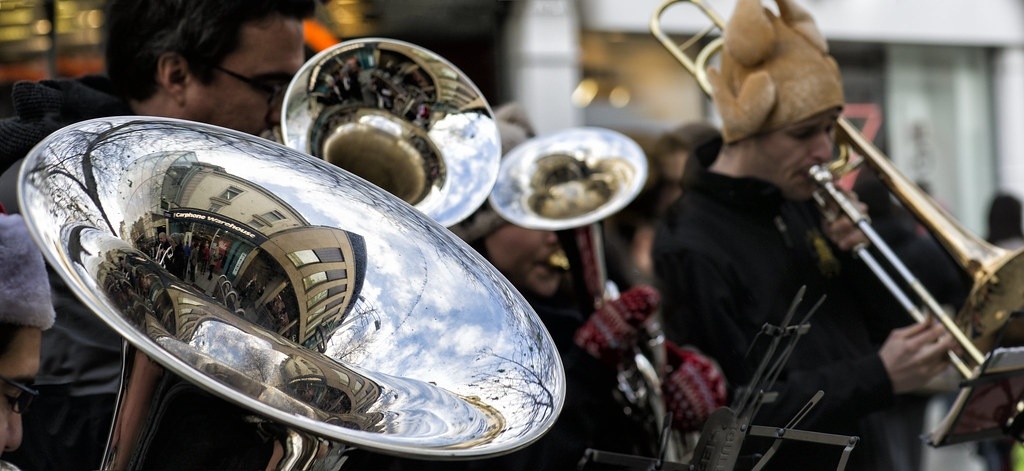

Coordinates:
[219,66,294,99]
[0,376,38,415]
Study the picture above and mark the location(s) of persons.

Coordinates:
[108,228,219,327]
[350,109,728,471]
[326,58,430,128]
[0,0,330,471]
[662,0,962,471]
[0,205,56,471]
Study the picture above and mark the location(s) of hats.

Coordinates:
[707,0,845,145]
[0,204,56,330]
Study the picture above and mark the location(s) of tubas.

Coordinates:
[259,38,502,227]
[16,115,566,471]
[488,127,667,435]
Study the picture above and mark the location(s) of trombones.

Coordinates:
[649,1,1023,428]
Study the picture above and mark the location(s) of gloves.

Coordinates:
[574,289,658,356]
[663,342,726,431]
